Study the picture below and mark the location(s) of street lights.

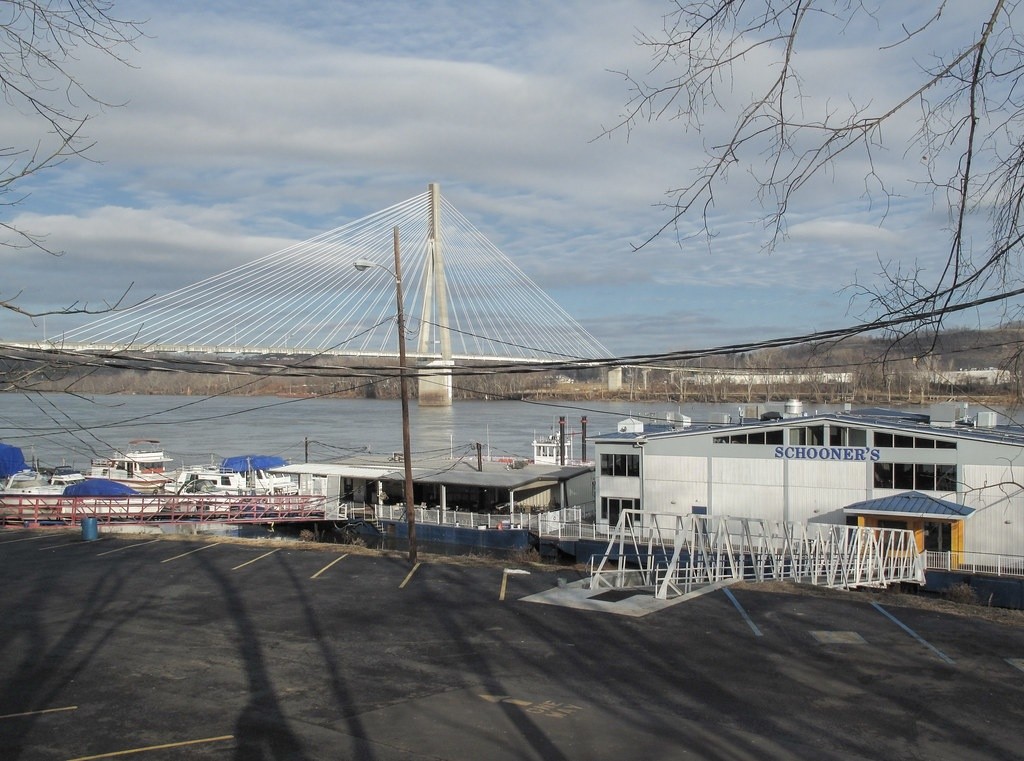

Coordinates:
[354,226,417,569]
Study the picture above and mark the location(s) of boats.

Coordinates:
[0,450,300,523]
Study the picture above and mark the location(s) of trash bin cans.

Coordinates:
[478,525,486,530]
[82,517,99,542]
[24,521,29,528]
